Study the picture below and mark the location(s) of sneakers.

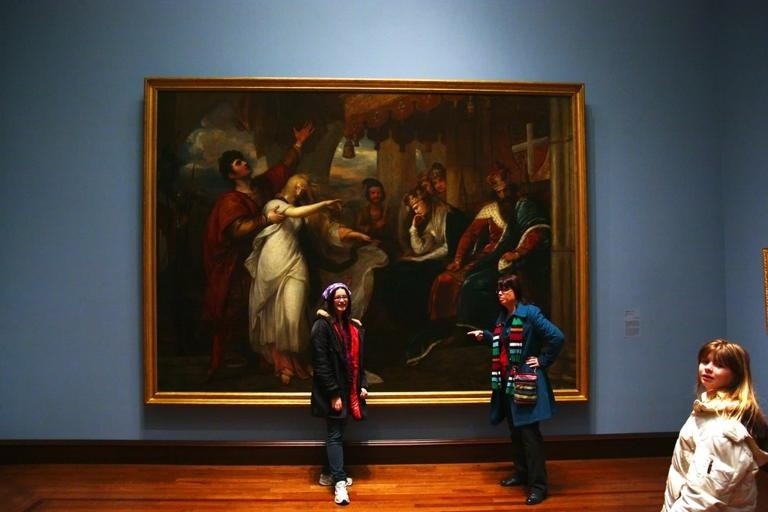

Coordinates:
[334,479,350,504]
[318,473,353,487]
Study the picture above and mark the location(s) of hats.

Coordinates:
[404,186,430,207]
[487,168,512,192]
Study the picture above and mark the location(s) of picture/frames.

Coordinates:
[140,74,590,403]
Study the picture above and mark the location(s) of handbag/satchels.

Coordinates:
[513,373,538,406]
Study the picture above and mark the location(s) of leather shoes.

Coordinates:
[526,491,548,505]
[500,476,525,486]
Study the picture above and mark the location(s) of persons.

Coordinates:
[662,338,766,511]
[468,276,565,505]
[310,282,370,505]
